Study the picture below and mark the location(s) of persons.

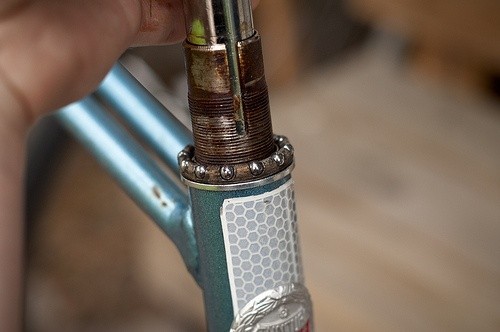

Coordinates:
[1,0,184,331]
